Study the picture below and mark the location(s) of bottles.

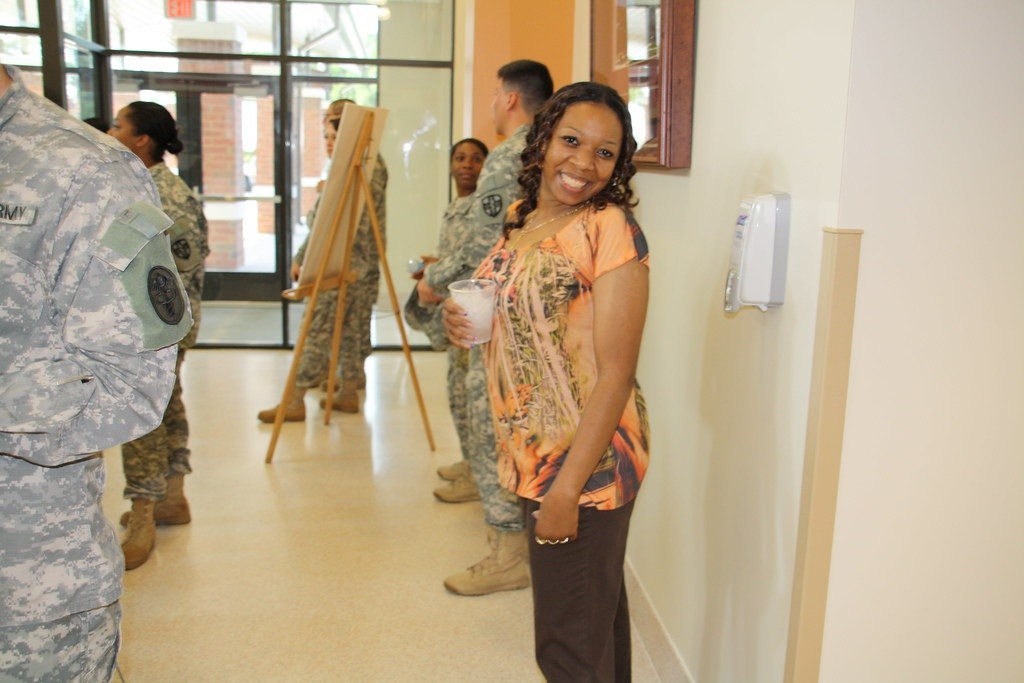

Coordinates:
[406,259,424,273]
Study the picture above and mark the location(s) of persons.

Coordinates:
[1,58,195,683]
[258,99,390,423]
[440,81,651,683]
[405,137,491,504]
[417,59,554,596]
[104,100,211,574]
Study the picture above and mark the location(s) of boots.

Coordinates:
[438,456,473,480]
[119,474,193,526]
[120,497,157,570]
[320,376,360,412]
[257,382,307,423]
[320,369,366,393]
[303,366,330,390]
[444,526,531,596]
[432,468,481,503]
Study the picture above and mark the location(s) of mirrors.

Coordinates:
[584,0,698,171]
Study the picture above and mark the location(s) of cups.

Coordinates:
[448,279,497,344]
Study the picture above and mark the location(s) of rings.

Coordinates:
[534,536,547,545]
[559,537,569,544]
[547,539,559,545]
